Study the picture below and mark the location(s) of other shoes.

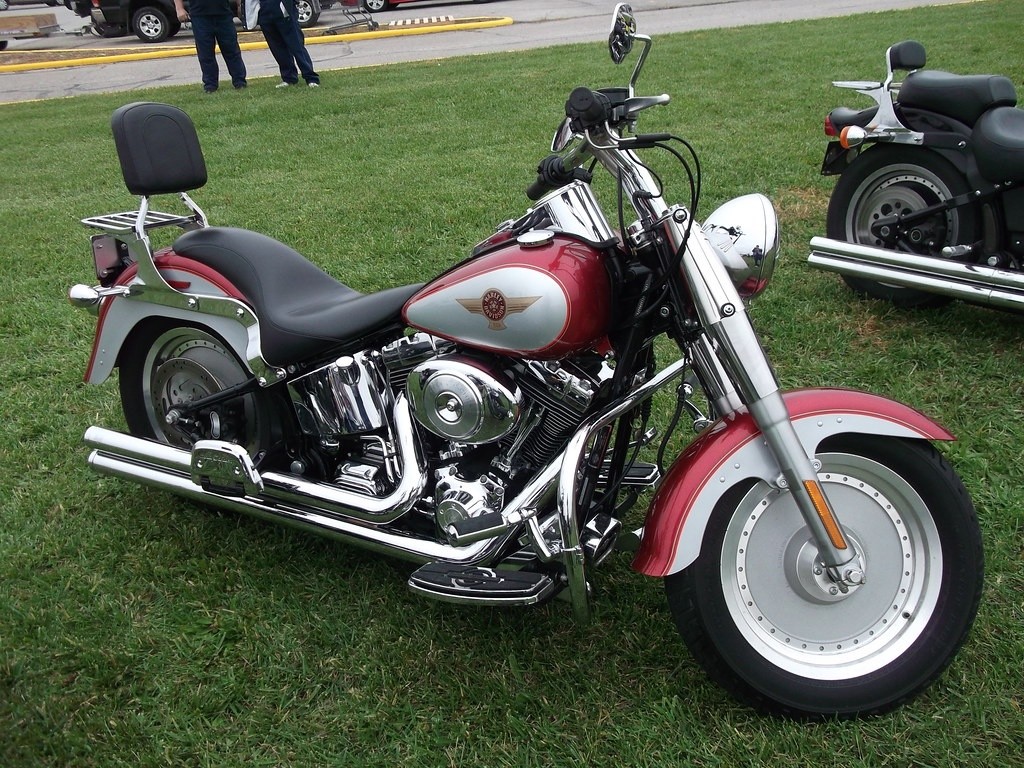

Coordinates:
[307,82,319,88]
[234,84,246,89]
[276,81,289,88]
[202,83,218,93]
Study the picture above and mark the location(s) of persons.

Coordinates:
[174,0,247,94]
[241,0,321,88]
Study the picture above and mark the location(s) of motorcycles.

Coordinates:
[69,3,982,721]
[806,41,1024,311]
